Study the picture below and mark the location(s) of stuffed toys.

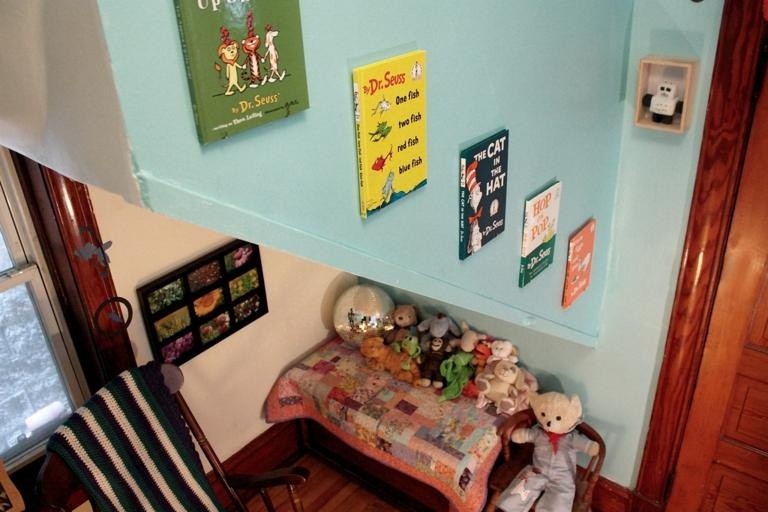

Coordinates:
[359,304,538,413]
[495,392,600,512]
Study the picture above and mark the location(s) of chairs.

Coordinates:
[486,410,607,512]
[33,359,312,512]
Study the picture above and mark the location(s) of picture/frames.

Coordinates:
[136,238,270,369]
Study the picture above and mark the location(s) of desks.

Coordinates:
[267,339,510,512]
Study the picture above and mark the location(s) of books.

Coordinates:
[174,1,309,144]
[518,177,562,287]
[457,126,509,260]
[351,49,425,218]
[561,216,595,309]
